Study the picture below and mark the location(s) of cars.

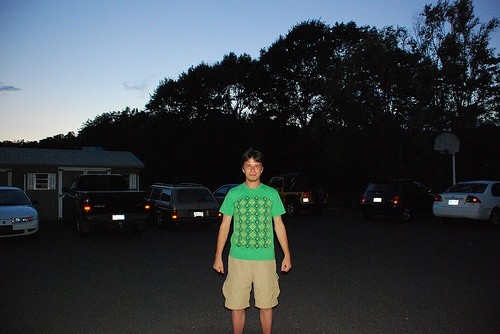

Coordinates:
[213,184,241,205]
[432,180,500,227]
[264,172,329,217]
[0,186,40,238]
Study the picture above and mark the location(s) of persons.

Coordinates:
[213,148,292,334]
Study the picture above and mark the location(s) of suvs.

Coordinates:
[360,176,435,223]
[144,182,223,230]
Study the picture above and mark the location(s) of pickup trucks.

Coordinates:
[63,174,149,238]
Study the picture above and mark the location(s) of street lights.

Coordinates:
[433,133,460,185]
